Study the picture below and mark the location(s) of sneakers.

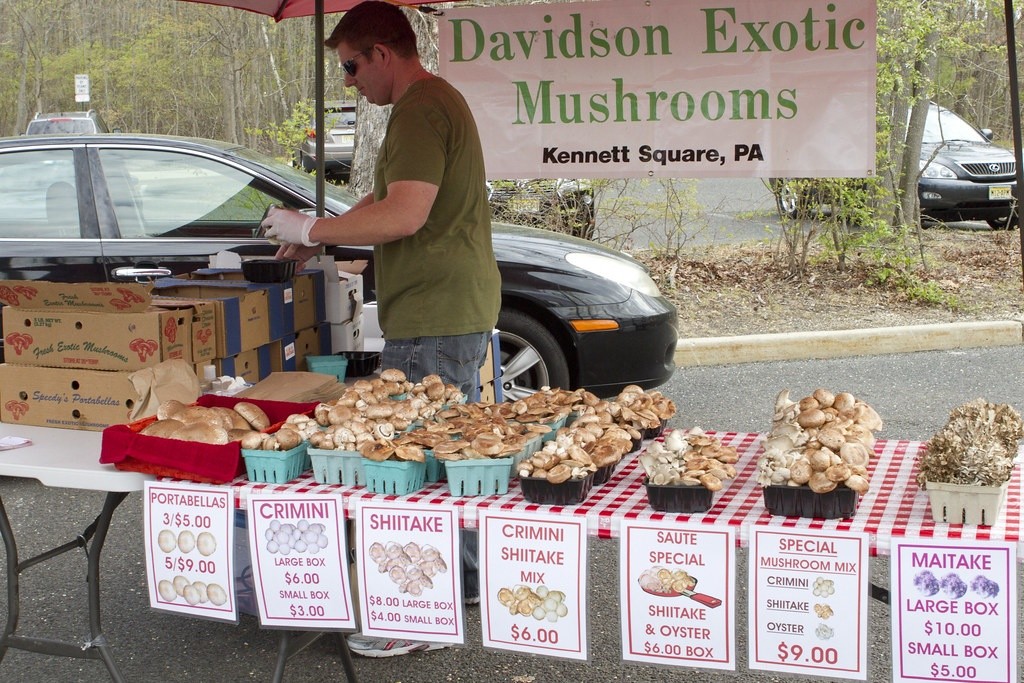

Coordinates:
[346,633,454,658]
[464,590,480,604]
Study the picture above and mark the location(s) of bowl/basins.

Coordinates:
[239,260,298,283]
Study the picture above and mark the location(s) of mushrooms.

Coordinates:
[638,566,696,593]
[157,530,228,606]
[812,577,834,639]
[265,521,330,554]
[369,541,448,595]
[498,584,568,622]
[139,369,677,484]
[757,388,882,494]
[639,425,740,491]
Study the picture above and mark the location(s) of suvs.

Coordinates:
[291,98,357,182]
[25,104,123,138]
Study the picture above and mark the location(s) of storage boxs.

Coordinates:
[0,258,1007,526]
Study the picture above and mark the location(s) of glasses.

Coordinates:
[342,48,372,77]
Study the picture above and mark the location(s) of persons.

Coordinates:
[261,1,501,657]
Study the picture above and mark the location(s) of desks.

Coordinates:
[0,421,161,683]
[158,429,1024,683]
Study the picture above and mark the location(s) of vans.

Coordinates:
[769,100,1018,230]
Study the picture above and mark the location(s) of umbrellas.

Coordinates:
[177,0,468,260]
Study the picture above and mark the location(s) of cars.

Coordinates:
[484,178,595,240]
[0,134,681,405]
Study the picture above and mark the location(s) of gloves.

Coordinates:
[261,208,321,247]
